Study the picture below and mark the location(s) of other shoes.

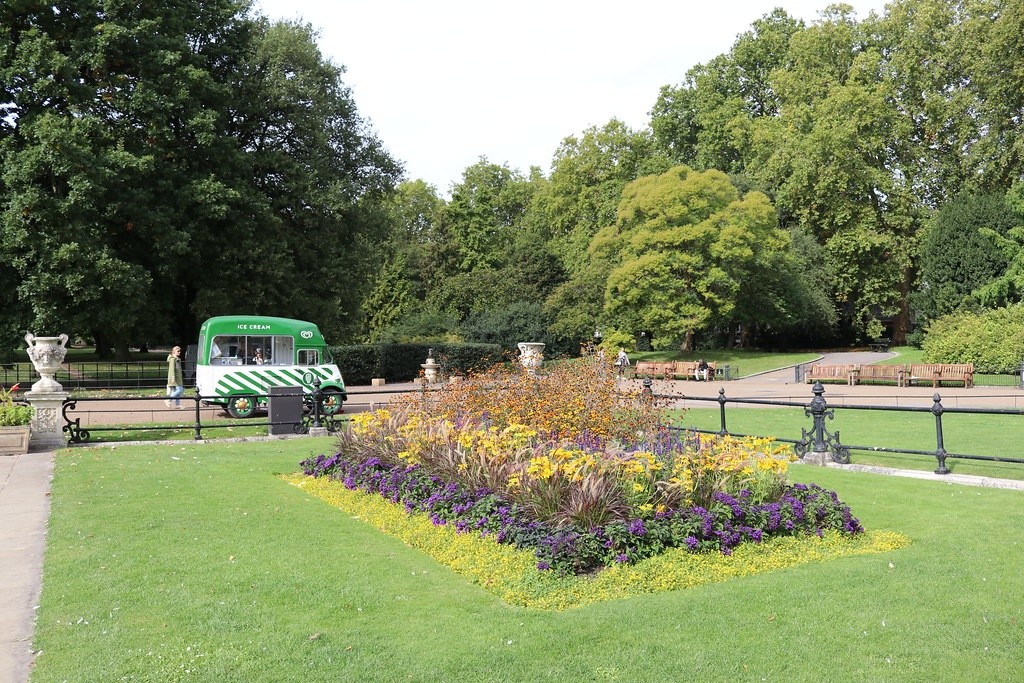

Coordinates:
[704,380,706,382]
[177,406,185,409]
[165,400,171,408]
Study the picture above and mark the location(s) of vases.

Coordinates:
[0,423,30,456]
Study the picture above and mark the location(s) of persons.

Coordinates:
[694,358,709,382]
[597,348,632,381]
[164,346,185,411]
[252,347,264,365]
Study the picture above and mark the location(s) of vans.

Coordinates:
[195,316,349,418]
[185,343,271,385]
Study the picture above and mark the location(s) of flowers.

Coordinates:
[0,383,35,426]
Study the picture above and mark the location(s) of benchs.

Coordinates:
[629,360,718,382]
[804,363,974,389]
